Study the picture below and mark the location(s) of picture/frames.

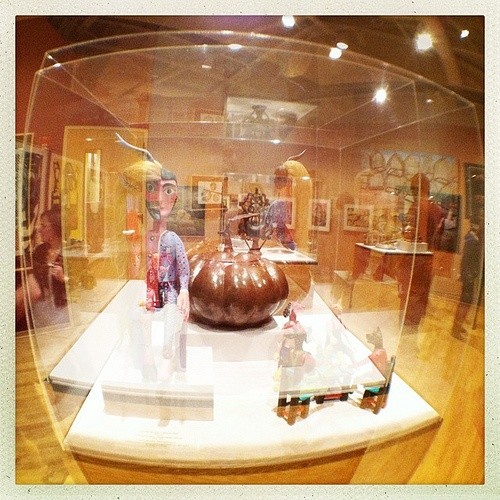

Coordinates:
[343,204,374,233]
[16,138,48,257]
[271,197,297,230]
[191,175,228,219]
[46,151,85,258]
[307,199,332,233]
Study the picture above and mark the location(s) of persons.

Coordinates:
[397,173,443,329]
[329,195,372,309]
[120,160,190,366]
[450,219,481,339]
[21,210,88,327]
